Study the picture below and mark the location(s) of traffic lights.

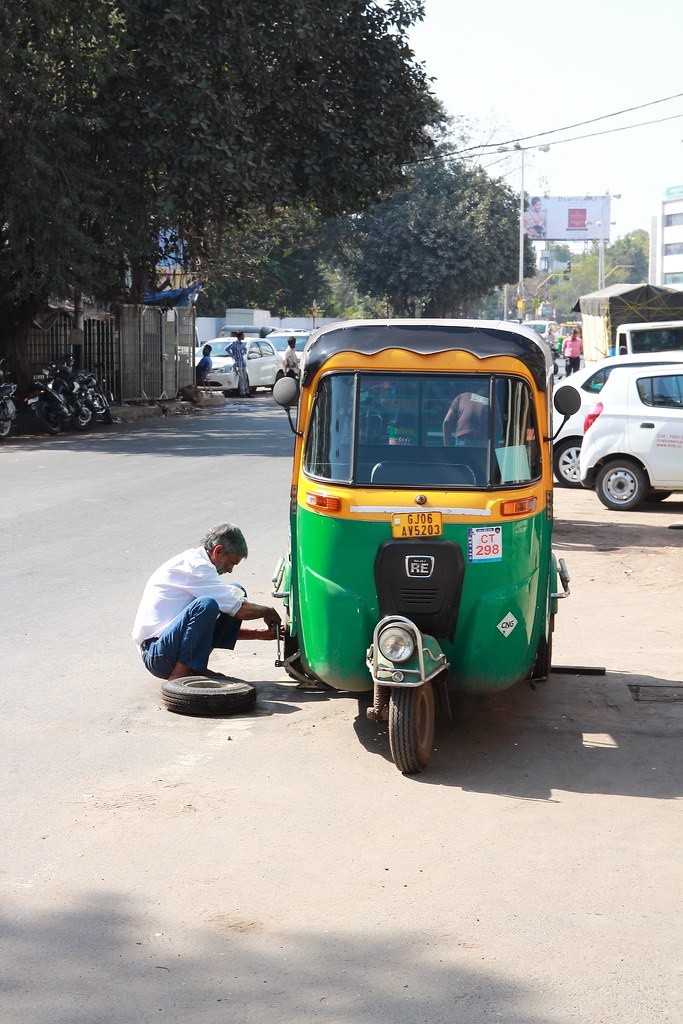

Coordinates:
[565,261,572,273]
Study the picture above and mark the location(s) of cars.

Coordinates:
[579,363,683,512]
[550,320,583,353]
[543,351,683,489]
[262,328,311,369]
[186,337,287,398]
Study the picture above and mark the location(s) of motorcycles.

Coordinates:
[0,358,20,441]
[23,351,115,436]
[273,317,582,776]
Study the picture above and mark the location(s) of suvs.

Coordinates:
[521,319,558,346]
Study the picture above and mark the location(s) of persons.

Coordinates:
[524,197,545,239]
[561,328,583,377]
[225,331,255,398]
[442,383,504,447]
[196,344,212,385]
[283,336,300,405]
[132,522,285,681]
[545,328,555,343]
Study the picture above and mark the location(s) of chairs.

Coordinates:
[196,365,213,396]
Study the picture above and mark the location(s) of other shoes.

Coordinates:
[241,394,255,398]
[202,669,225,677]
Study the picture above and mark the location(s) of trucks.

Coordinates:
[575,283,683,369]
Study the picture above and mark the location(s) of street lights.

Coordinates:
[584,189,623,289]
[584,220,617,290]
[498,142,552,322]
